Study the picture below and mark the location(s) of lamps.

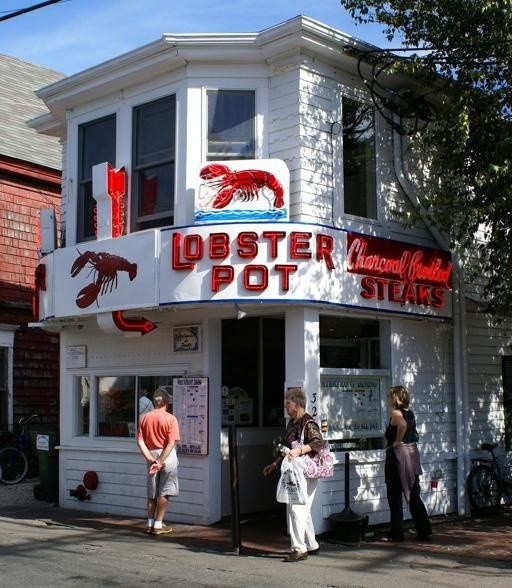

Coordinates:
[234,304,247,321]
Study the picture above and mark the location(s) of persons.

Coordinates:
[136,386,181,535]
[262,387,325,562]
[138,388,154,414]
[378,385,434,542]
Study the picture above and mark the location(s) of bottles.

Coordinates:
[277,443,290,456]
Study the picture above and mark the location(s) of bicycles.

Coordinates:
[1,413,58,485]
[467,441,511,513]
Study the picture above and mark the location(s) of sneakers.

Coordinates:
[285,551,308,561]
[146,521,172,535]
[308,547,319,554]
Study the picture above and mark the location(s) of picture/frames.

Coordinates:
[172,324,201,354]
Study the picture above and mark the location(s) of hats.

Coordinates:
[154,389,170,401]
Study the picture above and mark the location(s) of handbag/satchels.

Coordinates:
[291,441,333,478]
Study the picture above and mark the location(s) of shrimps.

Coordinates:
[201,164,284,208]
[71,249,137,309]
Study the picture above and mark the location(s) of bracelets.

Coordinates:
[273,462,278,469]
[298,446,303,454]
